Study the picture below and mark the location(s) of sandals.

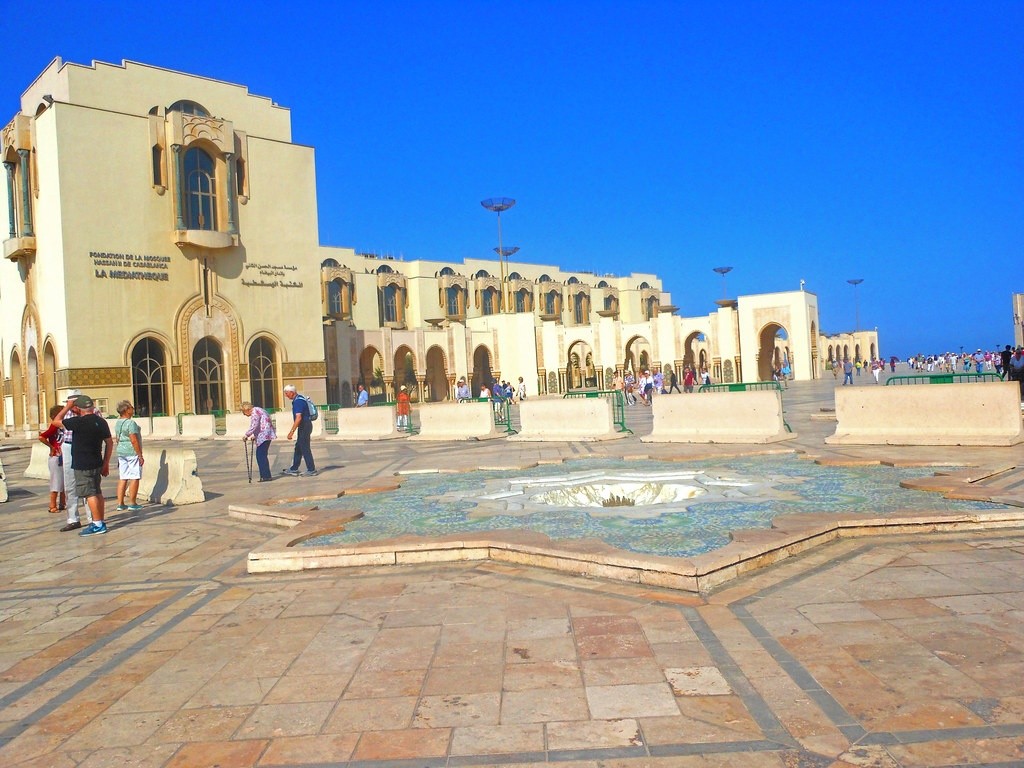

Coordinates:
[48,506,61,513]
[59,503,67,510]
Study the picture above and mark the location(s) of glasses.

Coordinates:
[129,406,133,409]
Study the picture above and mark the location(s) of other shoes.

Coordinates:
[257,477,272,482]
[60,522,81,531]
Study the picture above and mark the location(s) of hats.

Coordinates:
[401,385,407,391]
[73,396,93,408]
[62,395,80,403]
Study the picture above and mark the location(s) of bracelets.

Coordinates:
[138,457,143,459]
[291,430,294,432]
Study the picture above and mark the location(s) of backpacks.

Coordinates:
[295,397,318,421]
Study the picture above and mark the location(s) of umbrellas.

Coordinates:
[890,356,899,362]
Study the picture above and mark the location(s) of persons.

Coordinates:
[282,385,318,477]
[773,363,791,389]
[114,400,144,511]
[38,405,67,512]
[240,401,276,481]
[396,385,411,431]
[906,345,1024,402]
[831,357,896,385]
[356,384,369,407]
[58,389,102,532]
[52,395,113,537]
[479,378,516,422]
[612,367,712,406]
[455,380,469,403]
[516,377,526,401]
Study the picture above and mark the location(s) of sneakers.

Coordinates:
[300,469,318,476]
[79,522,107,537]
[116,505,128,510]
[283,468,298,476]
[128,504,143,511]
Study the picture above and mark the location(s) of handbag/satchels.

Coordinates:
[645,384,652,392]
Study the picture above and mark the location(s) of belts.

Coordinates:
[64,441,72,444]
